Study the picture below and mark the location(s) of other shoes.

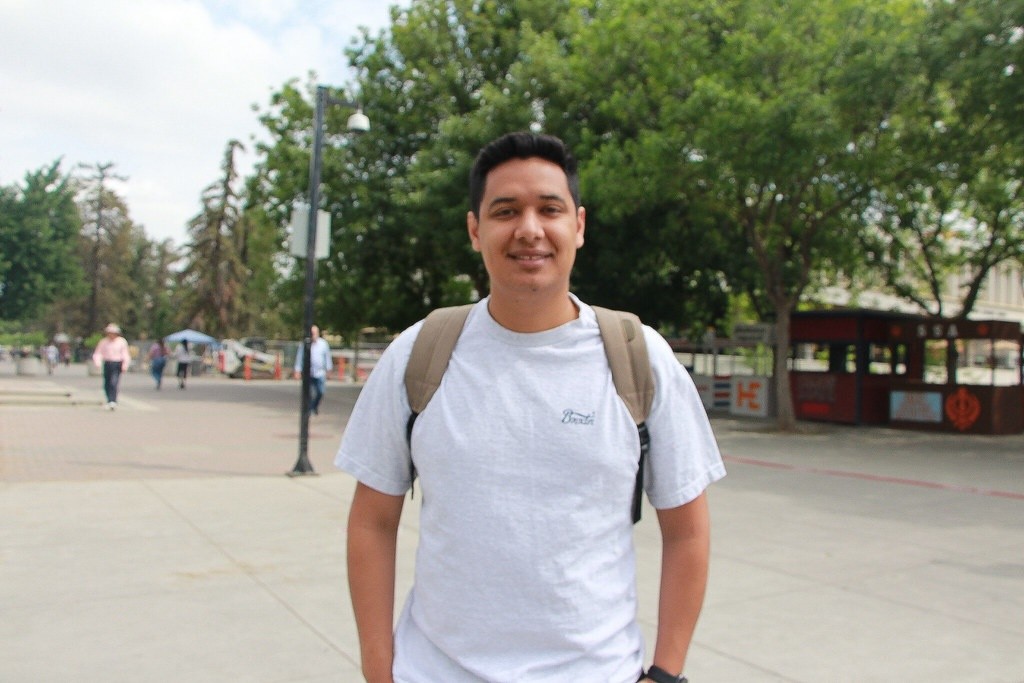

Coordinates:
[109,402,116,409]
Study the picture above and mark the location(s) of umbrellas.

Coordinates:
[168,329,214,343]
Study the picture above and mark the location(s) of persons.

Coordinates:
[333,134,726,683]
[145,337,168,389]
[172,340,191,388]
[293,325,333,419]
[10,342,75,375]
[92,322,129,410]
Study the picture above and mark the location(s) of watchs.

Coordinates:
[647,665,688,683]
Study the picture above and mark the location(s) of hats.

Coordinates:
[105,323,121,334]
